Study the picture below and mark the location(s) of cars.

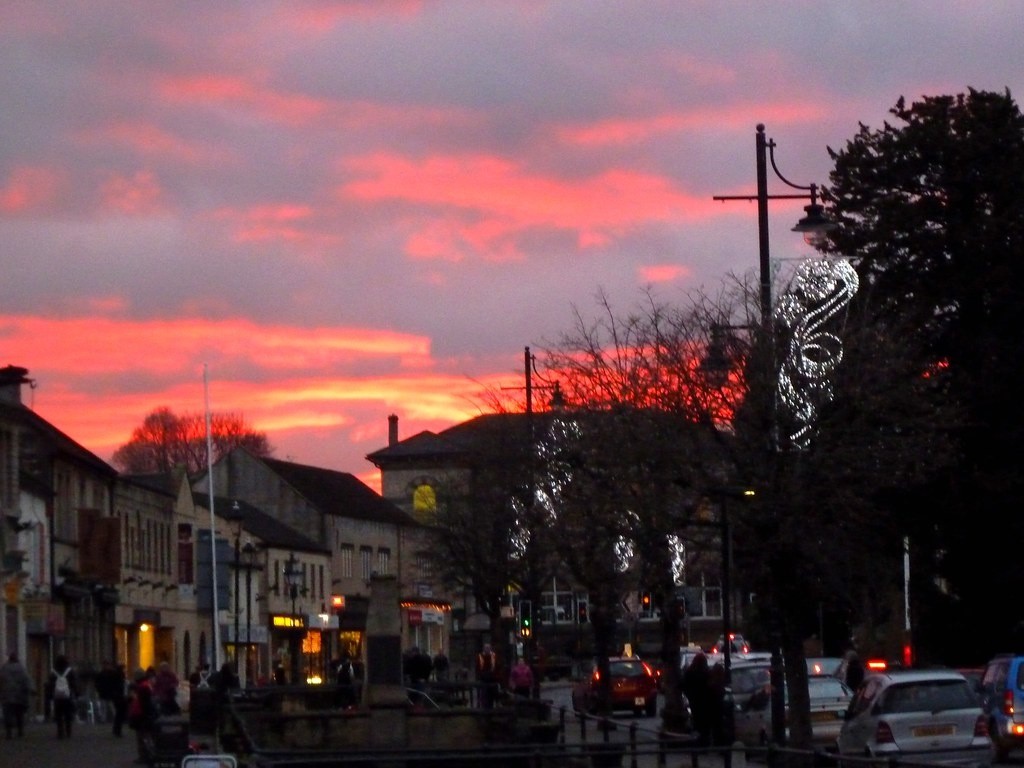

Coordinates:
[835,669,994,768]
[573,656,657,720]
[764,677,855,741]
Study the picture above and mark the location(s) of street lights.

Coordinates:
[722,487,756,665]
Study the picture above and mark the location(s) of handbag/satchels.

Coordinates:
[20,662,38,696]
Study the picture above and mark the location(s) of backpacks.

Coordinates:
[50,666,73,699]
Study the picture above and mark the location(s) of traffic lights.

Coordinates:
[578,600,588,624]
[518,599,532,639]
[642,590,651,613]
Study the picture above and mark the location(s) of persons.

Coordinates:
[844,651,865,690]
[745,685,771,712]
[476,643,499,706]
[95,657,182,762]
[191,664,216,687]
[0,653,34,739]
[407,647,432,691]
[681,655,725,745]
[536,642,546,681]
[509,657,533,698]
[432,648,448,683]
[568,640,584,681]
[48,655,78,739]
[336,658,356,704]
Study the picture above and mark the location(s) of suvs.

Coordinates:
[981,653,1024,750]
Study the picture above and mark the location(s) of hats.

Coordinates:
[198,681,210,691]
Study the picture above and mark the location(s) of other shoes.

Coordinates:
[66,732,72,738]
[112,730,123,738]
[17,731,25,737]
[4,733,13,740]
[133,755,150,765]
[57,733,65,739]
